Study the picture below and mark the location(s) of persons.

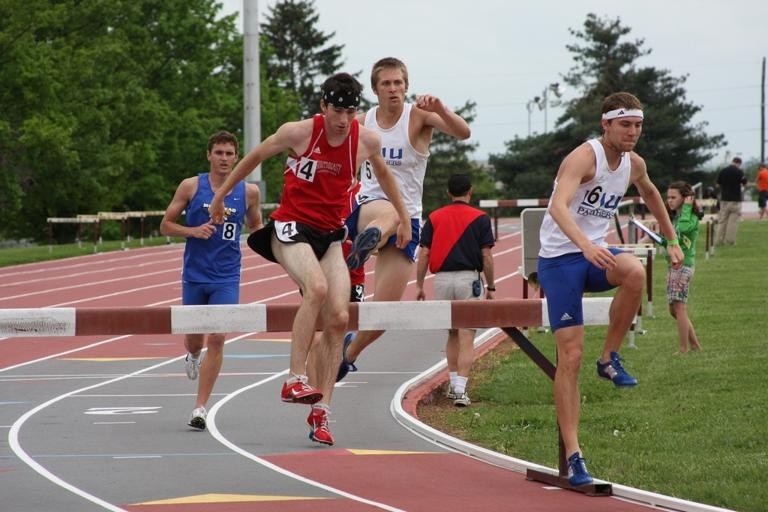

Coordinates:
[647,179,703,355]
[715,156,748,246]
[209,71,414,448]
[415,173,496,408]
[537,91,687,488]
[755,162,768,220]
[332,56,471,384]
[157,130,266,432]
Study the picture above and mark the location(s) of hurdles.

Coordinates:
[522,243,657,350]
[636,211,718,261]
[692,182,717,214]
[1,296,638,496]
[46,203,186,257]
[479,197,646,239]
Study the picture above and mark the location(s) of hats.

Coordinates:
[448,173,473,194]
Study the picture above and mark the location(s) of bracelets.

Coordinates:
[486,287,496,292]
[666,238,679,247]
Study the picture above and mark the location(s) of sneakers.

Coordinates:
[186,405,208,430]
[453,390,472,406]
[280,373,323,404]
[303,405,336,447]
[334,331,359,385]
[184,351,203,380]
[345,223,384,273]
[447,384,456,399]
[596,351,639,388]
[565,450,594,486]
